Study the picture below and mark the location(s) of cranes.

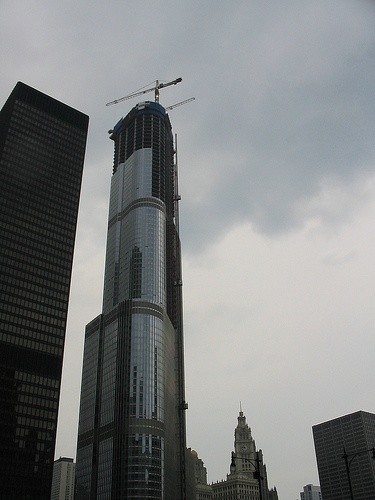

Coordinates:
[105,76,196,117]
